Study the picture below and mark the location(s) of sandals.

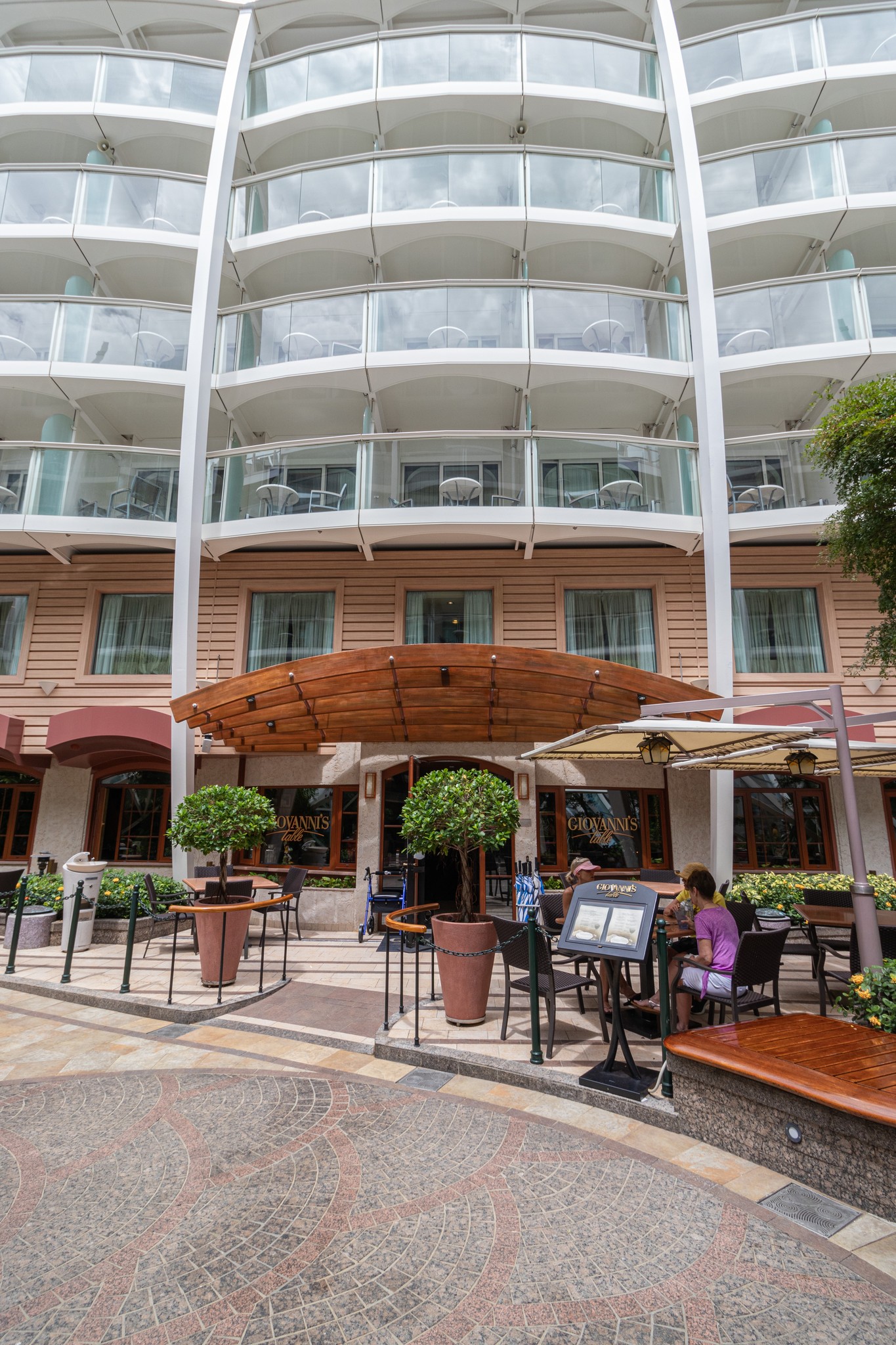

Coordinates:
[631,998,660,1015]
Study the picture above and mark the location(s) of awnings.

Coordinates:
[169,643,724,754]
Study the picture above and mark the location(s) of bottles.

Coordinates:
[684,898,694,920]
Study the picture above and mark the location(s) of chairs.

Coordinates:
[308,474,765,514]
[0,869,25,941]
[490,869,896,1060]
[78,474,162,521]
[142,864,309,958]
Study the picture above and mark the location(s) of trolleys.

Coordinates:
[358,866,406,944]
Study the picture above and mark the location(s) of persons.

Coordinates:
[631,869,747,1033]
[562,857,642,1016]
[663,861,728,1014]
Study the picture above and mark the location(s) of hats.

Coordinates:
[573,861,601,877]
[674,862,709,881]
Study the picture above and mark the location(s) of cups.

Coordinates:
[675,910,688,929]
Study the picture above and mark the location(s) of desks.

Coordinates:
[629,880,685,896]
[791,904,896,1007]
[182,875,279,960]
[598,480,643,510]
[256,483,300,516]
[0,319,770,367]
[0,486,18,514]
[555,914,703,1040]
[737,484,785,511]
[439,477,482,506]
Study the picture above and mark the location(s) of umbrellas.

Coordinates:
[662,711,896,777]
[513,857,545,925]
[516,684,883,976]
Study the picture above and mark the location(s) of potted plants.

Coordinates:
[397,768,522,1027]
[163,783,280,988]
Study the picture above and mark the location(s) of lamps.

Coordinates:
[517,772,529,802]
[861,678,884,695]
[636,679,709,767]
[38,681,59,696]
[197,681,275,727]
[783,747,819,775]
[365,772,377,800]
[440,667,448,671]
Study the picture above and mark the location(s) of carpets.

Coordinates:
[376,932,502,953]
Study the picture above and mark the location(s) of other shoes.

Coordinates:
[690,999,709,1015]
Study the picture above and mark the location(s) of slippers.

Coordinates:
[604,1007,613,1014]
[623,992,641,1006]
